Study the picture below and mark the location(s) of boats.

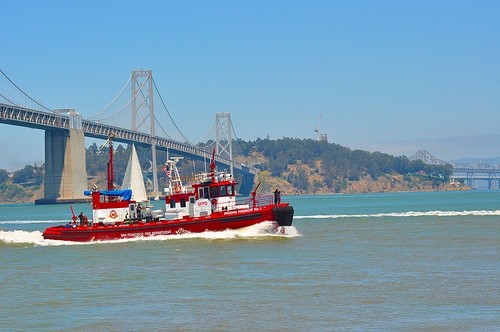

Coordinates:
[42,134,294,242]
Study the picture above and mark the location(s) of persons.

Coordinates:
[78,212,85,227]
[274,188,282,204]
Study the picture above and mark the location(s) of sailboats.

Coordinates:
[117,142,154,212]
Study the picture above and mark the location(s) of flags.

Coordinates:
[163,164,171,176]
